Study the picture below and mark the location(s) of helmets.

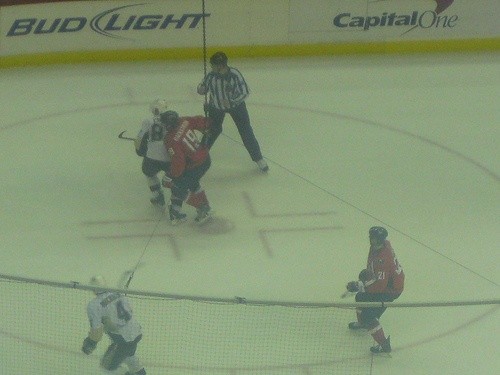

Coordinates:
[91,276,106,289]
[210,52,228,65]
[152,100,168,114]
[369,226,388,241]
[161,111,178,124]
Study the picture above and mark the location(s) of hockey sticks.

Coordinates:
[341,278,378,298]
[117,129,137,141]
[116,261,146,292]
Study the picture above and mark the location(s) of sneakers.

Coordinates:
[151,197,165,211]
[168,204,189,225]
[370,335,392,357]
[349,322,369,330]
[195,205,212,224]
[257,160,269,172]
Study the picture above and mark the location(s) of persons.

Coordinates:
[160,111,213,223]
[82,275,147,375]
[134,100,183,211]
[345,225,406,353]
[197,50,269,171]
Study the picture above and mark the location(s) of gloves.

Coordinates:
[359,270,368,281]
[346,281,360,292]
[82,338,97,355]
[162,173,174,189]
